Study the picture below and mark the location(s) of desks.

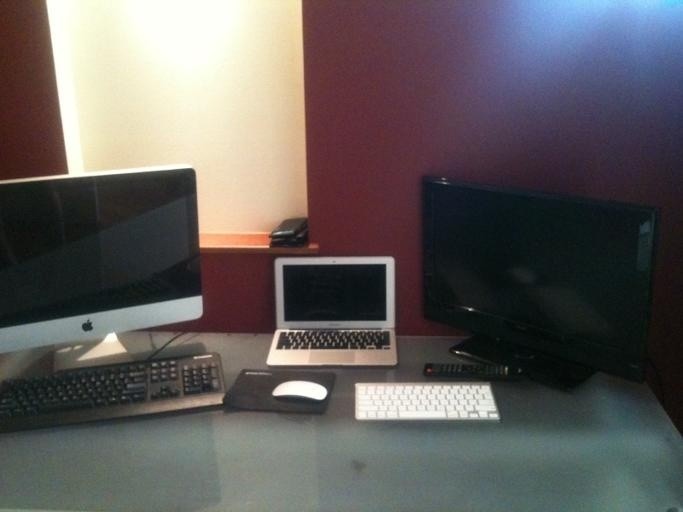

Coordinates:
[0,331,682,512]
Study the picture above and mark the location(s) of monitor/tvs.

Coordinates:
[0,166,204,373]
[421,176,662,394]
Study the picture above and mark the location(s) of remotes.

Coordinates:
[423,362,510,380]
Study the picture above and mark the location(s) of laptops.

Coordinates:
[266,255,399,366]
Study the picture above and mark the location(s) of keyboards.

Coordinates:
[353,381,501,423]
[0,353,228,434]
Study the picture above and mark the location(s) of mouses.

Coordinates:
[271,380,328,401]
